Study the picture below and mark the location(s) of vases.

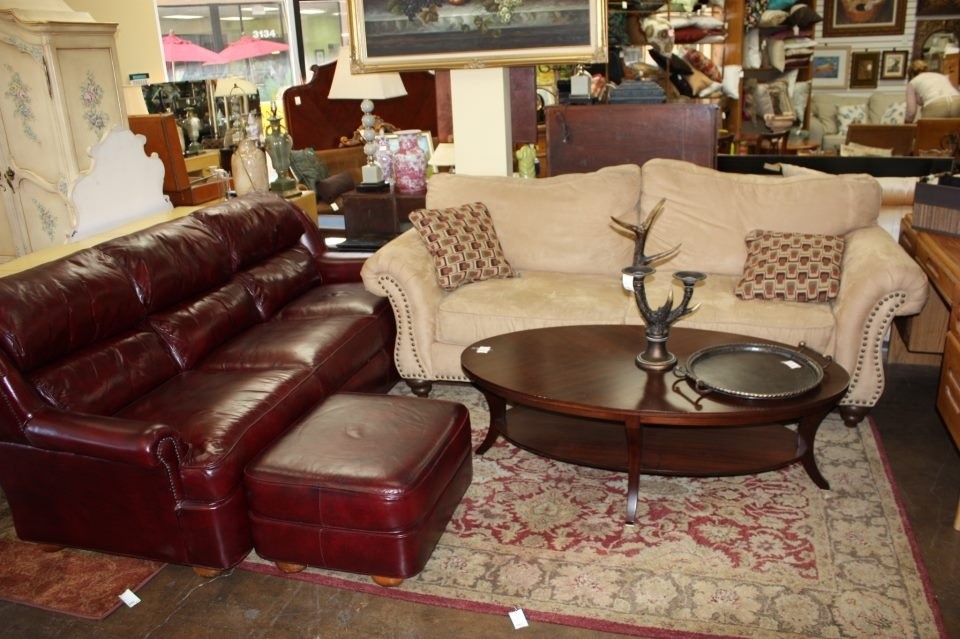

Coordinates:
[394,129,426,191]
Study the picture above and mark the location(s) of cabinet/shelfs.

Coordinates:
[887,213,960,530]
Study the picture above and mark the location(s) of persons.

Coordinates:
[904,60,960,123]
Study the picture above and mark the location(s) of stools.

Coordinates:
[243,395,473,589]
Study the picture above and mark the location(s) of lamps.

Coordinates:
[326,46,407,190]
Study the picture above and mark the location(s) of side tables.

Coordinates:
[289,190,319,229]
[342,189,430,242]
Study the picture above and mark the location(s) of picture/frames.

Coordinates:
[810,0,909,90]
[348,0,609,75]
[374,131,439,182]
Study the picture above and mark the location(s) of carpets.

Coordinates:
[0,532,169,620]
[234,378,945,639]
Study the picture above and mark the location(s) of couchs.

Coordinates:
[0,191,405,576]
[359,172,926,432]
[809,91,922,151]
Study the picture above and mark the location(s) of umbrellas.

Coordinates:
[163,29,219,81]
[204,32,289,79]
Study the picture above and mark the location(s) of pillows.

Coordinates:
[685,48,724,98]
[409,201,514,291]
[882,103,920,124]
[835,103,867,134]
[734,229,845,303]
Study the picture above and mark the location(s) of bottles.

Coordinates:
[376,136,391,184]
[392,129,427,194]
[231,139,269,196]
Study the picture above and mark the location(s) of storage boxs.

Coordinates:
[127,113,233,206]
